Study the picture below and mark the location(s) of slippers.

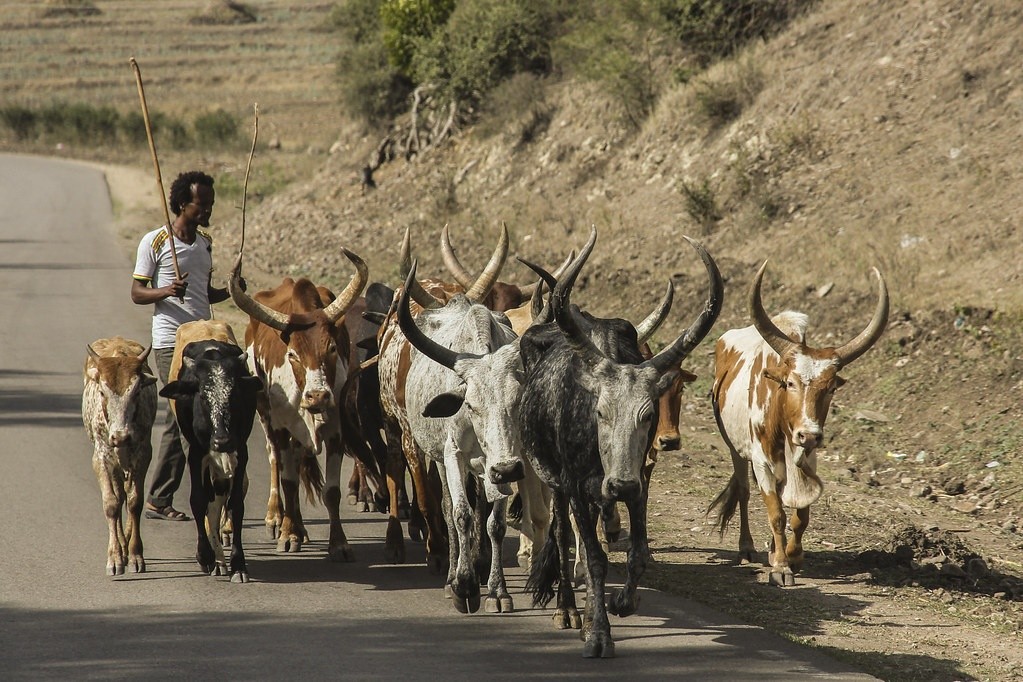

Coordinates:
[146,505,186,520]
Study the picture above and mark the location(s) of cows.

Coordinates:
[82,223,890,657]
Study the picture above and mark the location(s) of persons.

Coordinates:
[131,171,247,521]
